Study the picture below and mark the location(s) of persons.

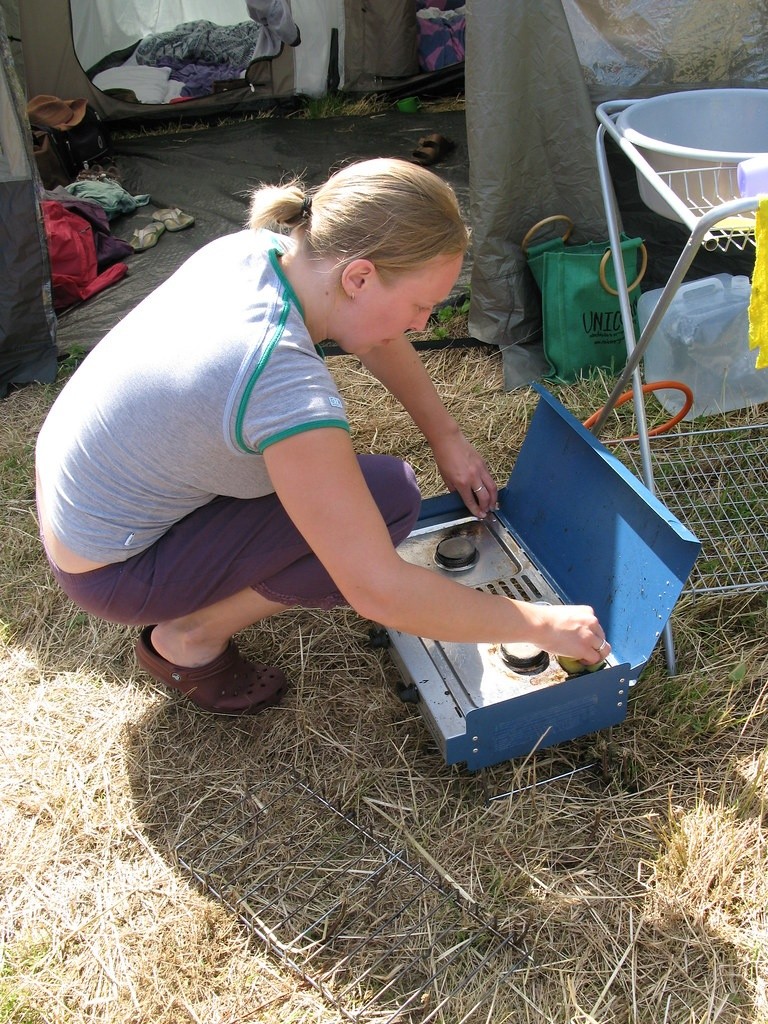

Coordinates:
[36,159,611,717]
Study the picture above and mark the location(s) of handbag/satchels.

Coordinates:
[522,215,647,386]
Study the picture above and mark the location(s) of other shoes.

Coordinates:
[74,164,123,221]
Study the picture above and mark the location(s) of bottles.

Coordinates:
[637,274,768,421]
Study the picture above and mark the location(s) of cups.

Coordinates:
[397,96,423,114]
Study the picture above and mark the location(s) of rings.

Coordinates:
[474,484,484,493]
[596,639,606,652]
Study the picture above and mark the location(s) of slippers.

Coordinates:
[152,208,194,231]
[135,624,287,715]
[129,222,165,251]
[411,133,455,166]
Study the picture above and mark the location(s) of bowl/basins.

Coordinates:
[619,89,768,228]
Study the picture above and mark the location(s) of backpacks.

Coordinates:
[48,104,113,182]
